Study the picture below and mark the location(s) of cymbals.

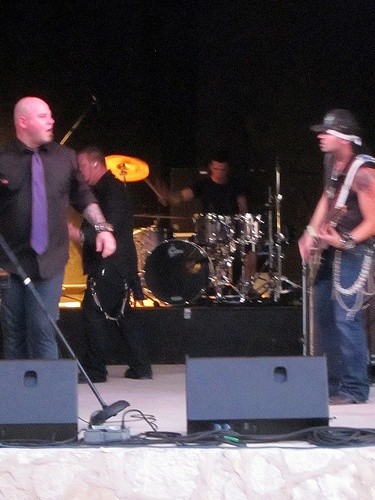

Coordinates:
[105,154,150,183]
[255,203,284,210]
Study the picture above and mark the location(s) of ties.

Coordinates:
[29,148,50,255]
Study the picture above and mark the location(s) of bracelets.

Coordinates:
[342,233,356,249]
[94,223,114,234]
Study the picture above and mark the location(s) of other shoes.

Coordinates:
[327,392,355,405]
[78,373,90,383]
[125,368,152,378]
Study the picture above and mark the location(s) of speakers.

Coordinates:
[0,359,79,444]
[184,357,330,443]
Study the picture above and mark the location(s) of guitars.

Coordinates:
[307,205,347,286]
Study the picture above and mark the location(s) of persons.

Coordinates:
[153,153,249,288]
[0,97,116,360]
[67,147,152,383]
[298,110,375,405]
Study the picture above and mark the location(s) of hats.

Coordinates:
[310,109,360,134]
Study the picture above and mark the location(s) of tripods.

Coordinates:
[250,159,301,302]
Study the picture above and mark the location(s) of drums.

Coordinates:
[195,213,234,250]
[231,213,263,246]
[132,226,162,289]
[144,240,214,306]
[207,250,233,287]
